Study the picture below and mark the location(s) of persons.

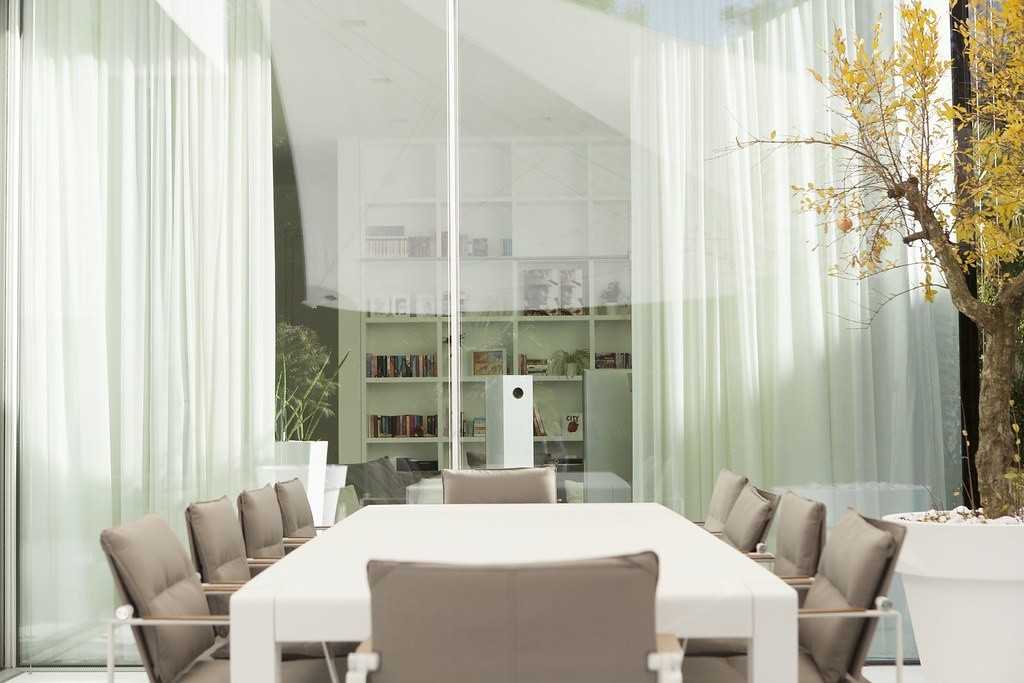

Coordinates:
[524,269,585,317]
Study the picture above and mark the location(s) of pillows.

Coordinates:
[336,455,440,505]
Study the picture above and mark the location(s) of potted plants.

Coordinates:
[704,0,1024,682]
[276,323,351,527]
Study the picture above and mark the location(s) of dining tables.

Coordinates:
[405,467,631,504]
[230,502,798,683]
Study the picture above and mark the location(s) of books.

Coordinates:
[366,267,512,316]
[369,402,548,437]
[365,353,632,377]
[365,225,513,258]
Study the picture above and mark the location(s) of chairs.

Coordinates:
[100,466,907,683]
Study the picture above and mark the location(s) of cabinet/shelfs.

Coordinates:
[336,136,633,463]
